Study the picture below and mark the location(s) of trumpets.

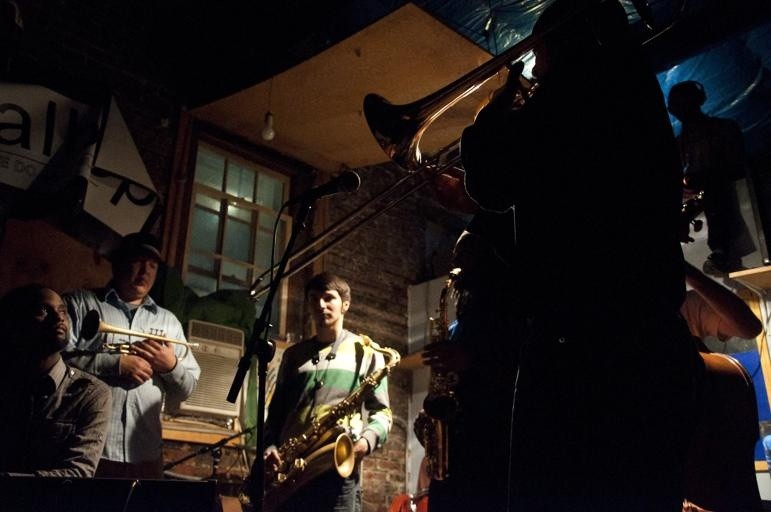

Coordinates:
[74,310,200,360]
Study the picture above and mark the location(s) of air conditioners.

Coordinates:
[178,318,245,420]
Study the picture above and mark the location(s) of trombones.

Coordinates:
[249,0,686,299]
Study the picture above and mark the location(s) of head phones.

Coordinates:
[668,80,706,115]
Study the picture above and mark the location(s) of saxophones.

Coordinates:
[422,268,463,485]
[240,334,401,510]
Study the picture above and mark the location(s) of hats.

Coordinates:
[111,232,170,273]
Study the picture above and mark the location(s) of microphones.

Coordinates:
[285,169,361,207]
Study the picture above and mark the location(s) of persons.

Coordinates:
[3,284,112,477]
[258,271,394,511]
[427,1,715,511]
[681,259,762,341]
[65,233,201,478]
